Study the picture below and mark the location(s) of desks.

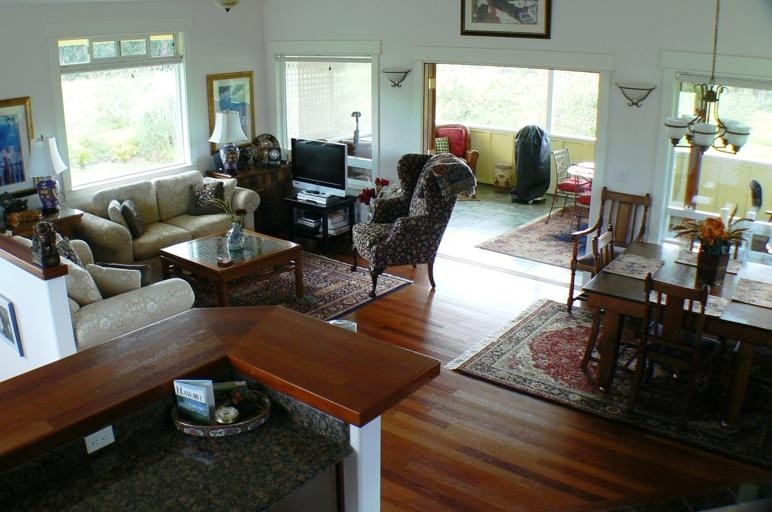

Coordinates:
[5,208,84,243]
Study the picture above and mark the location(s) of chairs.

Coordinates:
[12,235,197,354]
[544,147,594,239]
[627,273,728,423]
[566,186,650,312]
[349,154,470,298]
[431,124,480,195]
[580,225,658,377]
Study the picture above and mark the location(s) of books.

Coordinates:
[173,379,216,426]
[298,210,350,236]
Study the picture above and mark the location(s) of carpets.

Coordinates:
[476,203,590,271]
[456,192,480,202]
[446,299,772,470]
[159,250,413,321]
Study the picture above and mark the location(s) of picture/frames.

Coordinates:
[459,0,551,40]
[206,71,257,156]
[1,293,25,357]
[0,96,43,201]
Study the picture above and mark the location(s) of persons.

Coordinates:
[0,145,22,186]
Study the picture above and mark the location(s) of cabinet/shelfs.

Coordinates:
[283,193,358,250]
[206,165,293,234]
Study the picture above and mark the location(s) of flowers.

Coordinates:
[358,178,389,222]
[197,186,247,222]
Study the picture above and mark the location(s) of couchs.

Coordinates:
[63,170,261,283]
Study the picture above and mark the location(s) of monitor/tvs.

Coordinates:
[290,137,348,199]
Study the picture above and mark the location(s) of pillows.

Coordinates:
[56,233,153,304]
[433,137,450,155]
[203,176,238,212]
[190,181,225,216]
[108,200,130,230]
[122,198,146,238]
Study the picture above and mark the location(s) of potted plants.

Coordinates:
[0,192,28,228]
[671,204,755,284]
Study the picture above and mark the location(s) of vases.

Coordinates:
[227,221,245,251]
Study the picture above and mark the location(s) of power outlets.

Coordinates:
[84,425,117,455]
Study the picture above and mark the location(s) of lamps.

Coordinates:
[208,111,248,175]
[27,134,68,214]
[664,0,752,155]
[381,67,412,89]
[616,82,657,108]
